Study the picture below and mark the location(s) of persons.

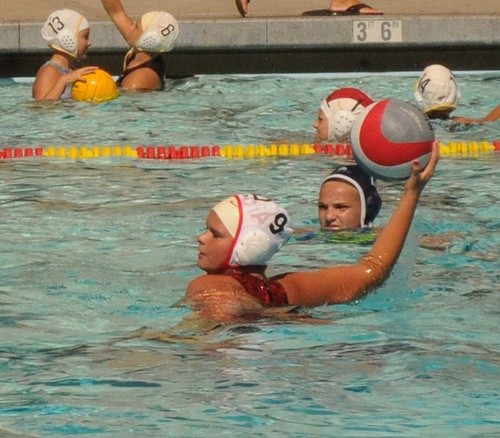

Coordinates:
[414,64,499,122]
[32,9,98,100]
[186,140,441,308]
[313,87,374,142]
[102,0,178,90]
[235,0,385,18]
[318,165,382,230]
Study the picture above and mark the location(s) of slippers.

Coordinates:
[324,2,384,16]
[234,0,250,20]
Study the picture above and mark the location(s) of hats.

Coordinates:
[413,63,460,117]
[136,10,179,52]
[320,165,384,227]
[224,193,294,269]
[320,88,377,143]
[41,9,84,58]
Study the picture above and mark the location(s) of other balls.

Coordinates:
[71,69,117,101]
[350,98,435,180]
